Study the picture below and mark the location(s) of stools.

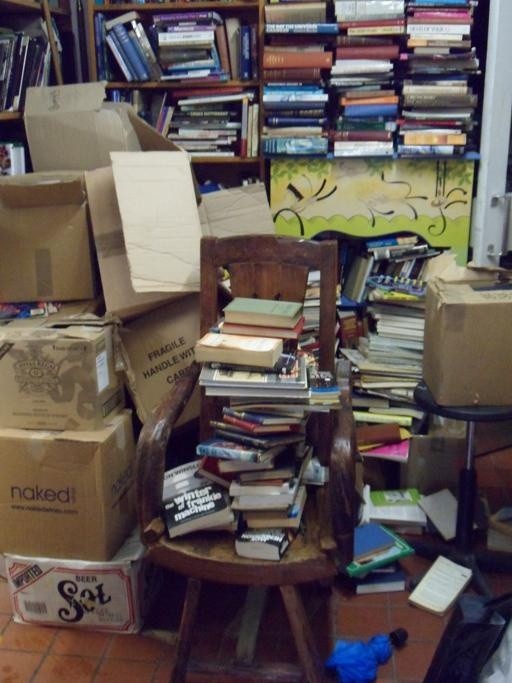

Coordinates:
[397,380,511,604]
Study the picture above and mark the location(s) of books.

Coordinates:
[0,17,60,177]
[407,556,474,616]
[163,235,452,595]
[263,1,482,157]
[94,9,262,194]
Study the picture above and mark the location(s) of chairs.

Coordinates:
[134,231,361,683]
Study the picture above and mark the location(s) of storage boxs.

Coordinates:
[0,409,136,561]
[0,290,129,433]
[0,525,154,636]
[1,78,279,320]
[423,268,512,411]
[111,292,224,435]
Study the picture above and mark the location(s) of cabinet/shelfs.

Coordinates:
[0,2,78,132]
[85,0,266,166]
